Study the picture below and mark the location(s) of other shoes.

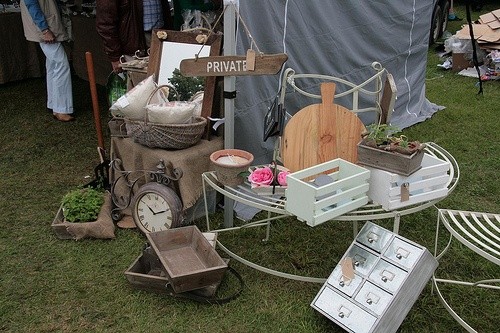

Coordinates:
[53,112,76,122]
[448,15,464,21]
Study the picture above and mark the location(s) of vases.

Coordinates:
[123,224,231,298]
[210,149,254,187]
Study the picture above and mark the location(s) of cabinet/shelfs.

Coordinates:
[310,221,439,333]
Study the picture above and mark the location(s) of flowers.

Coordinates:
[236,165,291,187]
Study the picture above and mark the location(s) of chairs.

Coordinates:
[261,61,386,243]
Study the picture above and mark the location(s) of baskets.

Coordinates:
[124,85,206,149]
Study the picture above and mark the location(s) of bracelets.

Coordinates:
[42,28,49,33]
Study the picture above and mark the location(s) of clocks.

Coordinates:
[131,182,182,236]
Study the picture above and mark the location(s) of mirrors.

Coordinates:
[148,28,222,141]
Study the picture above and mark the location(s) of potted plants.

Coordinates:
[50,188,116,241]
[356,125,425,176]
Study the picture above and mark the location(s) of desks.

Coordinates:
[108,136,223,229]
[431,210,500,333]
[202,142,460,282]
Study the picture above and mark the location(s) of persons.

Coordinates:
[20,0,75,122]
[448,0,463,21]
[96,0,172,92]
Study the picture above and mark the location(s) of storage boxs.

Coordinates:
[284,156,371,227]
[363,153,450,212]
